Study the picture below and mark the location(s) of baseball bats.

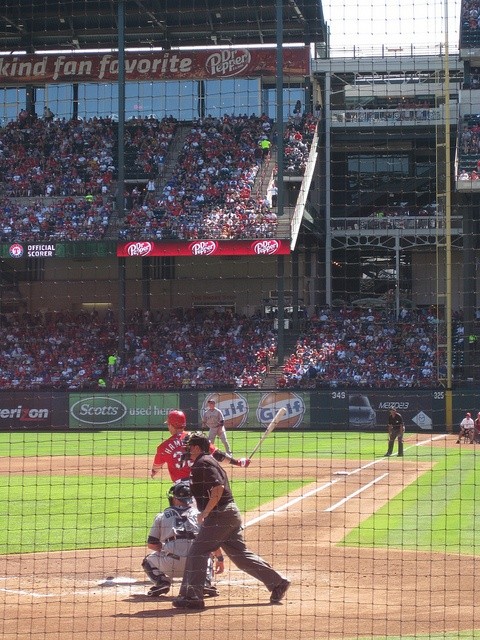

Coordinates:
[249,408,286,459]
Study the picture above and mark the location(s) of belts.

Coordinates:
[164,534,196,543]
[203,500,234,510]
[181,477,192,481]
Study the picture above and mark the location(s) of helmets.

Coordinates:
[183,432,209,461]
[388,406,397,411]
[208,398,216,404]
[163,411,186,429]
[174,482,194,505]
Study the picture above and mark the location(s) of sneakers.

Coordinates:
[148,581,170,597]
[203,582,220,596]
[270,579,291,601]
[172,595,204,609]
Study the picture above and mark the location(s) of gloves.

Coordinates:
[237,457,251,468]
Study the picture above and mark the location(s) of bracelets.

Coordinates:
[230,458,238,465]
[217,555,224,562]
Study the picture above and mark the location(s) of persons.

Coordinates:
[0,308,118,390]
[457,323,464,336]
[176,113,278,178]
[279,306,446,390]
[142,484,225,596]
[283,99,323,176]
[123,113,176,177]
[119,178,278,240]
[385,407,405,456]
[346,100,432,121]
[459,121,480,180]
[360,210,439,229]
[151,410,253,482]
[469,332,480,350]
[174,432,290,608]
[0,106,117,243]
[474,412,480,443]
[203,400,232,456]
[118,306,279,390]
[456,413,474,444]
